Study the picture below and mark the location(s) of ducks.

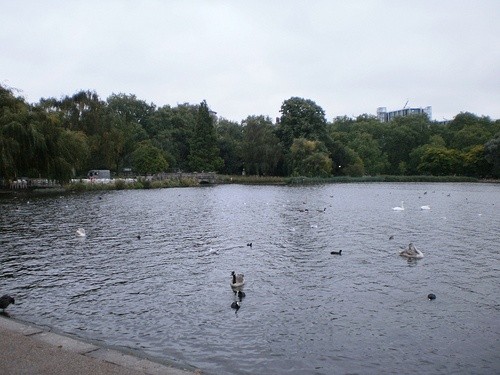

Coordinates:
[15,187,496,288]
